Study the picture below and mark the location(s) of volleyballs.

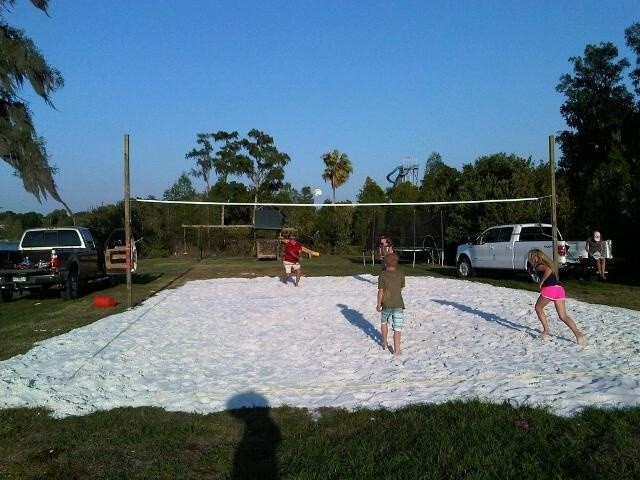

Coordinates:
[314,189,322,197]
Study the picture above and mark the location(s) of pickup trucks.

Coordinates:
[0,226,137,301]
[455,222,613,280]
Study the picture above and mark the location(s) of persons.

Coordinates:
[281,233,304,287]
[585,229,607,280]
[378,236,394,267]
[527,249,584,346]
[375,252,406,356]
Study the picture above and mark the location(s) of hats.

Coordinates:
[593,231,601,242]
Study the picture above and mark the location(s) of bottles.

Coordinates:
[38,259,42,269]
[24,256,29,269]
